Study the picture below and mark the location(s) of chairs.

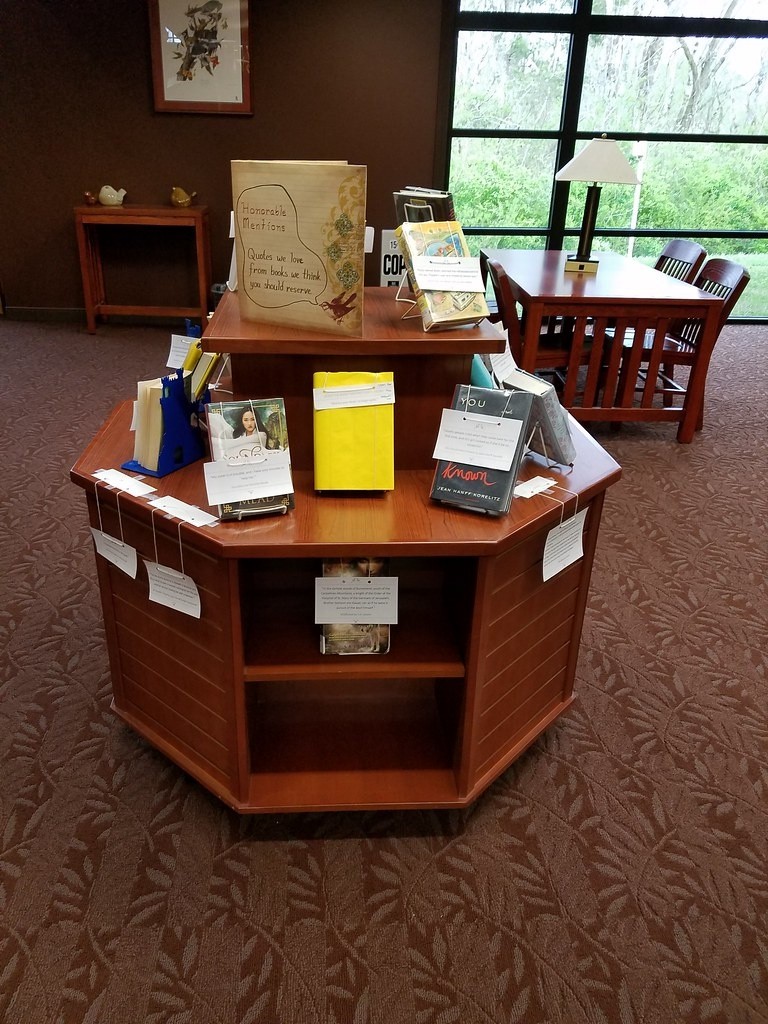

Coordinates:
[591,257,750,433]
[605,239,707,329]
[485,260,612,407]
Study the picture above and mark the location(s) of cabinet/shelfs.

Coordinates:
[69,287,622,814]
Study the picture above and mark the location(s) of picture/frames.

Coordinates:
[146,0,255,116]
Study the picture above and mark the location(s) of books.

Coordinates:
[132,311,231,472]
[392,185,490,329]
[313,372,394,491]
[429,384,535,516]
[203,398,296,521]
[230,159,367,339]
[315,558,398,655]
[502,367,577,467]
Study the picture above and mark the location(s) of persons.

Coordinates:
[356,558,384,577]
[233,406,268,449]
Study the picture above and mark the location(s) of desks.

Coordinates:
[73,201,209,334]
[480,249,725,443]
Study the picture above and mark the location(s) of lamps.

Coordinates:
[555,132,642,273]
